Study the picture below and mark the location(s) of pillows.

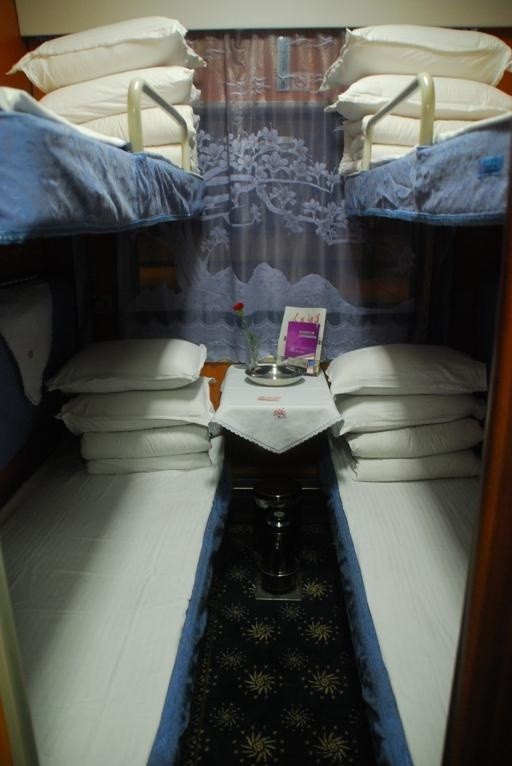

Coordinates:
[323,340,487,401]
[320,73,511,119]
[314,23,512,91]
[44,333,210,392]
[55,374,218,435]
[4,13,207,99]
[330,392,488,439]
[24,64,200,122]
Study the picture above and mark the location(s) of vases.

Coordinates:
[243,334,261,370]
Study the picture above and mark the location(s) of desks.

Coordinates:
[217,358,340,600]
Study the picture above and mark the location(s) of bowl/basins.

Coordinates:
[244,364,306,386]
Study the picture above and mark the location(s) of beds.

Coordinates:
[0,87,208,255]
[4,356,233,765]
[317,441,512,765]
[336,105,511,229]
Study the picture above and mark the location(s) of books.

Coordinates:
[281,320,320,376]
[277,305,326,377]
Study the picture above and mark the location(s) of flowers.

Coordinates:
[233,301,255,353]
